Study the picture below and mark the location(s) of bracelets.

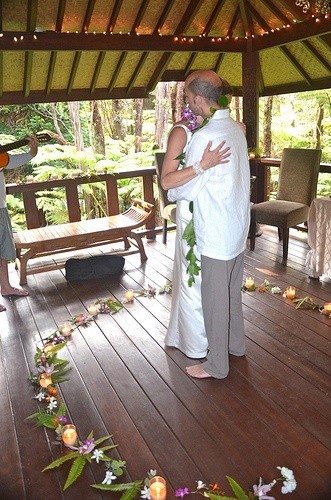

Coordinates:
[193,161,205,176]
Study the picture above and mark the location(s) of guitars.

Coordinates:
[0,132,53,174]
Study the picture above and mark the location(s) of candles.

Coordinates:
[40,373,52,388]
[61,321,71,337]
[125,288,134,302]
[324,302,331,315]
[148,476,167,500]
[88,303,98,316]
[246,277,254,289]
[61,425,77,447]
[286,286,295,299]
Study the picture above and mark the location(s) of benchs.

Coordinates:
[12,198,154,286]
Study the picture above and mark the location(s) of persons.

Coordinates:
[167,69,252,379]
[160,76,247,359]
[0,134,39,312]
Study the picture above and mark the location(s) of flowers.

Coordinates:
[181,106,197,131]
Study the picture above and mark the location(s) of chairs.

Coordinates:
[250,148,322,259]
[154,152,176,244]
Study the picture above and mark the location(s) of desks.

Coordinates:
[305,197,331,284]
